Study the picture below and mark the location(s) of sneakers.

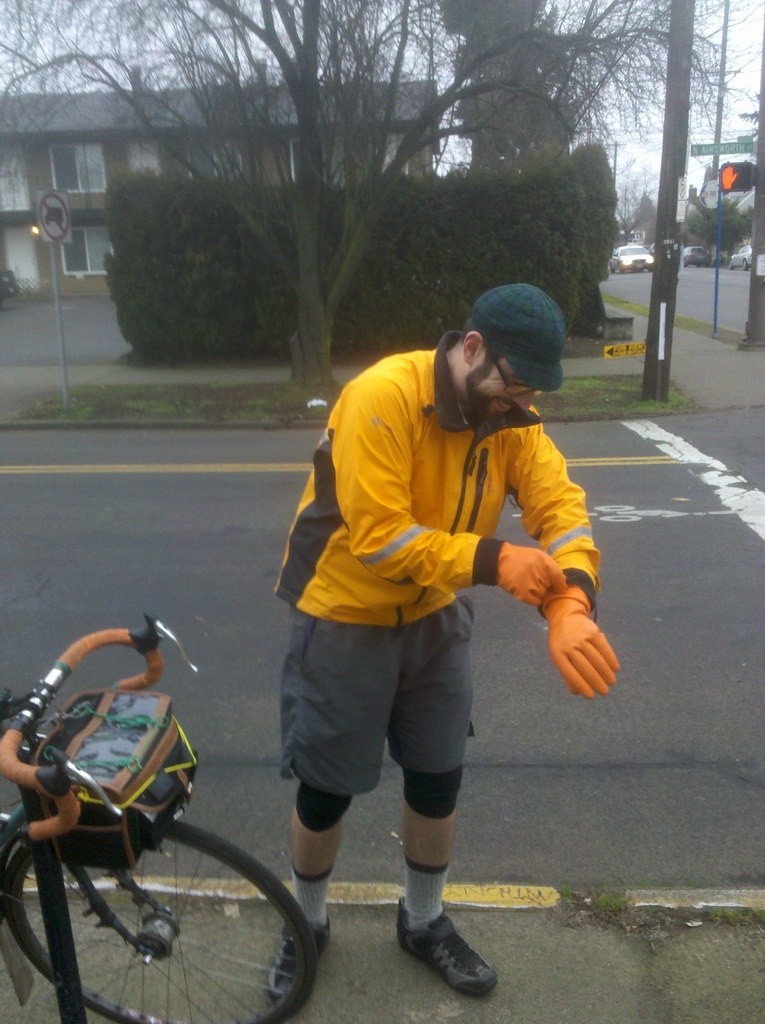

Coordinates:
[266,913,330,1005]
[395,895,498,997]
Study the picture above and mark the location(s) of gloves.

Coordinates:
[541,581,620,700]
[495,541,568,606]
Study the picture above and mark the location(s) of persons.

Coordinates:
[265,282,619,1008]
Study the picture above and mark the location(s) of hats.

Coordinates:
[471,283,567,393]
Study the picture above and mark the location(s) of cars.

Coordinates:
[632,239,641,243]
[683,246,710,267]
[609,245,654,274]
[636,241,645,246]
[641,245,651,251]
[628,238,633,242]
[648,242,655,257]
[627,242,635,246]
[0,270,20,303]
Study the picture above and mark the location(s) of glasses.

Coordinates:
[487,347,543,398]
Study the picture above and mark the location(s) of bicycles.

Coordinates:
[0,614,317,1024]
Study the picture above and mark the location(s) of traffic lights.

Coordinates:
[720,162,754,193]
